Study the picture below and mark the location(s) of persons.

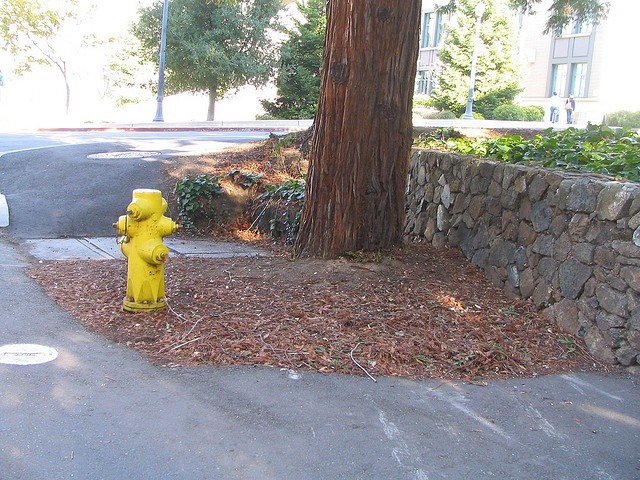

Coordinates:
[565,94,576,124]
[549,90,559,123]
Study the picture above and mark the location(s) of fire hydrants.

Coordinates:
[110,187,184,316]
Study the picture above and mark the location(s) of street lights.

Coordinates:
[459,1,487,120]
